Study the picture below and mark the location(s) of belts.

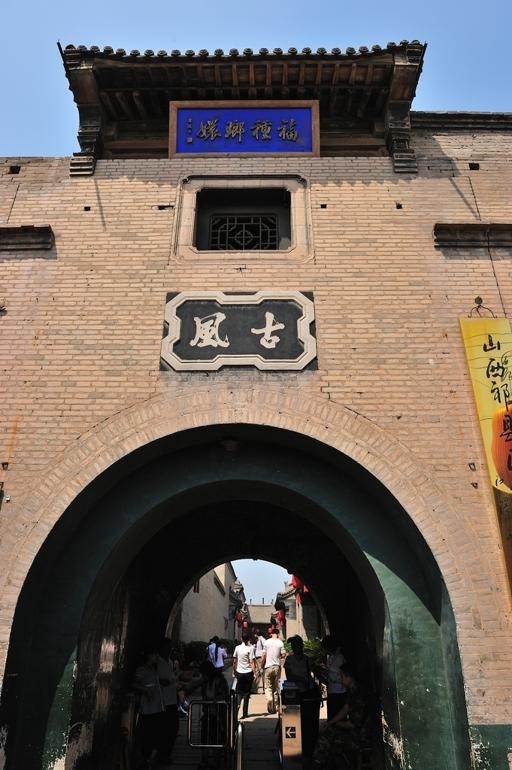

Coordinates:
[255,656,262,659]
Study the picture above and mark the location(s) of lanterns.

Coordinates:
[235,572,310,641]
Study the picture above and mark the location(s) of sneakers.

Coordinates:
[267,701,280,714]
[240,715,248,720]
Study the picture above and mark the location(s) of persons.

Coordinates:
[129,627,371,769]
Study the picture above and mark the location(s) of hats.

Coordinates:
[287,635,303,643]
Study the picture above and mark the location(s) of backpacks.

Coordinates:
[130,644,173,694]
